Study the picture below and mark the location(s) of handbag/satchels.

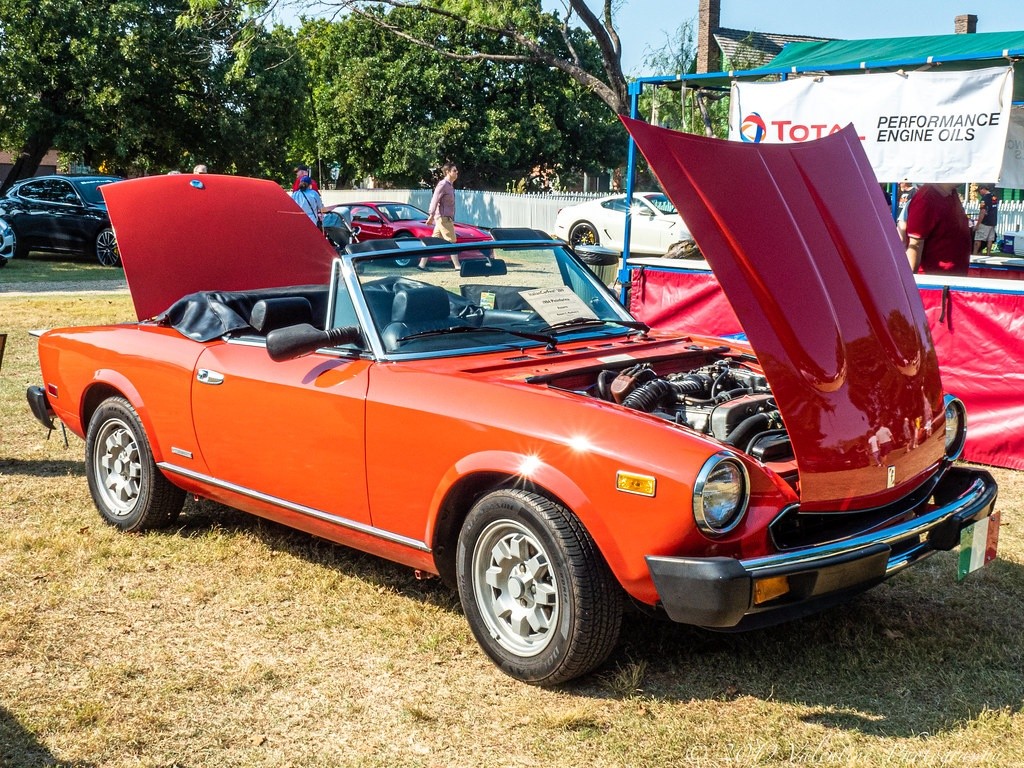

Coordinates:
[317,220,323,229]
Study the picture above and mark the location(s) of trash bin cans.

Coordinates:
[574,242,621,304]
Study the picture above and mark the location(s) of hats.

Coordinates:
[295,165,308,172]
[975,184,988,191]
[300,176,312,184]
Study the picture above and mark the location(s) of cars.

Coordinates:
[0,172,125,268]
[0,218,18,267]
[554,191,701,261]
[25,114,998,687]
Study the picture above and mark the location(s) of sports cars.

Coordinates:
[320,200,496,267]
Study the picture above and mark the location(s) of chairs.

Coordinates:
[249,298,313,337]
[381,286,472,352]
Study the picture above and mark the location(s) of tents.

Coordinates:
[619,30,1024,274]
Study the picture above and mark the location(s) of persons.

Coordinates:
[855,421,896,468]
[193,165,207,174]
[292,165,321,196]
[416,163,461,273]
[879,183,918,218]
[973,184,998,256]
[291,176,326,226]
[905,183,971,277]
[902,415,922,453]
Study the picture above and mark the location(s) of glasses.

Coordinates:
[195,171,207,174]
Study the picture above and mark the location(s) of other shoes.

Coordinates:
[417,266,432,272]
[454,268,462,272]
